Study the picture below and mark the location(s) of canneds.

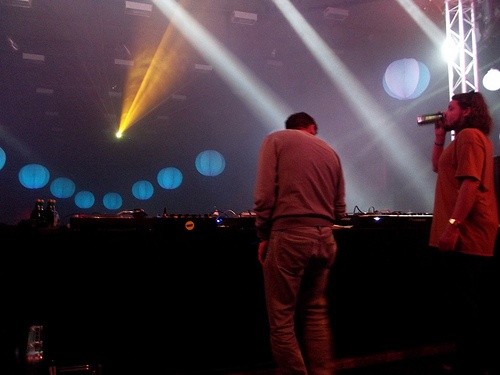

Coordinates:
[35,199,44,220]
[46,199,57,215]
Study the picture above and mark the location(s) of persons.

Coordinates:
[253,112,348,375]
[428,91,500,375]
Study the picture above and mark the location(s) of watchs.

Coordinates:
[448,217,460,227]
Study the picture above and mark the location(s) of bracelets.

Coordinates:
[434,140,445,148]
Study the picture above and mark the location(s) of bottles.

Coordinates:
[31,199,60,227]
